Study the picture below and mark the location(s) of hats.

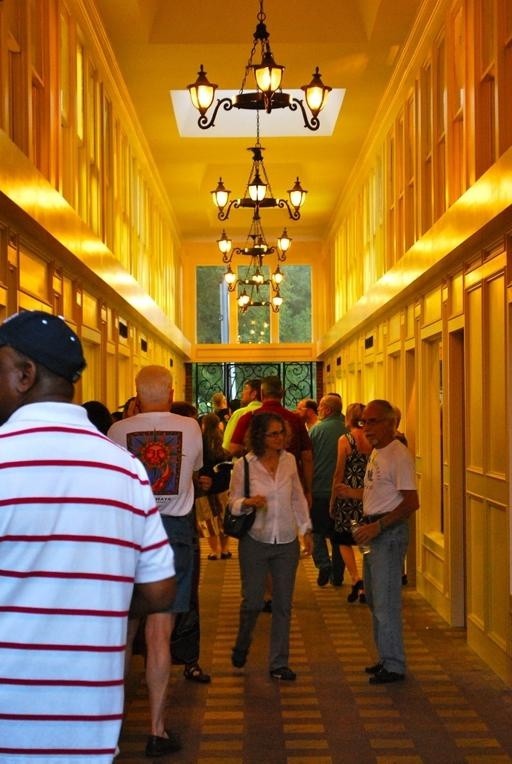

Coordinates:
[0,310,86,383]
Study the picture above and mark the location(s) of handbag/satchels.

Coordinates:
[223,502,257,539]
[195,495,220,538]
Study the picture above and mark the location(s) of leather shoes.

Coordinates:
[144,729,183,757]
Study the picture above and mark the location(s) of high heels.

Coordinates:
[347,579,366,604]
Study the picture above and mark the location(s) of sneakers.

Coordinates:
[365,663,406,685]
[270,665,297,682]
[231,651,247,668]
[206,550,232,561]
[316,561,345,587]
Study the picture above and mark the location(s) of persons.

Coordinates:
[82,365,420,760]
[1,311,178,764]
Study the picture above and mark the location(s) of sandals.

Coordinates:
[183,662,211,684]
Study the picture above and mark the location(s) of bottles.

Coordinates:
[350,519,371,556]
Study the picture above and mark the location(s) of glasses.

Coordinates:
[262,426,289,437]
[356,418,388,430]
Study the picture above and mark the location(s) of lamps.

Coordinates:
[224,256,285,294]
[185,0,335,131]
[211,82,309,223]
[238,283,281,314]
[217,208,292,271]
